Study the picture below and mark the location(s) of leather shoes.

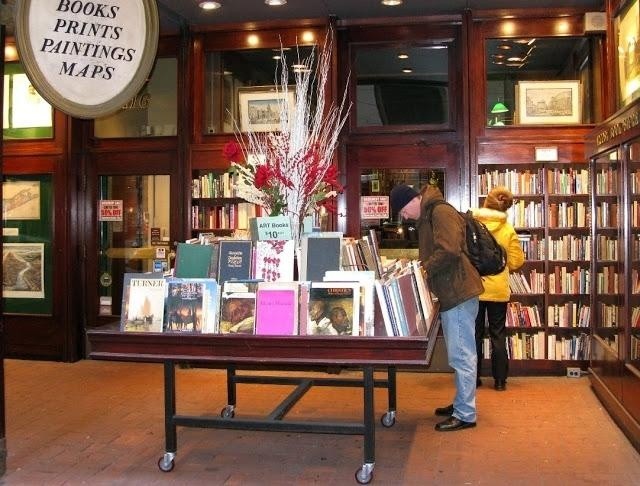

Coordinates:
[476,378,482,388]
[435,404,453,416]
[435,416,476,431]
[495,379,506,391]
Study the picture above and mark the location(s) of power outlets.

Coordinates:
[567,367,581,378]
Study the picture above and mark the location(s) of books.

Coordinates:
[595,166,640,360]
[478,168,590,361]
[119,171,437,343]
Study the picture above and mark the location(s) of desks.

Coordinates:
[86,300,440,485]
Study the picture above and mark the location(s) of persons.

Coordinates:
[390,181,486,433]
[465,186,526,392]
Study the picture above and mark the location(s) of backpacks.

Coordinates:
[429,200,507,276]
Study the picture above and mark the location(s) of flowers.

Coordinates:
[220,22,357,235]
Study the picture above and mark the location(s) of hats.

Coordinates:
[390,186,419,212]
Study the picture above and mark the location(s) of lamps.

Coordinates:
[488,97,510,126]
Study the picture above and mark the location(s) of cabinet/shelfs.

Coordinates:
[472,128,592,378]
[77,142,186,360]
[341,126,471,256]
[186,142,334,240]
[0,58,79,361]
[587,102,640,454]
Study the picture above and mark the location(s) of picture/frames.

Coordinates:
[518,80,582,127]
[233,86,298,134]
